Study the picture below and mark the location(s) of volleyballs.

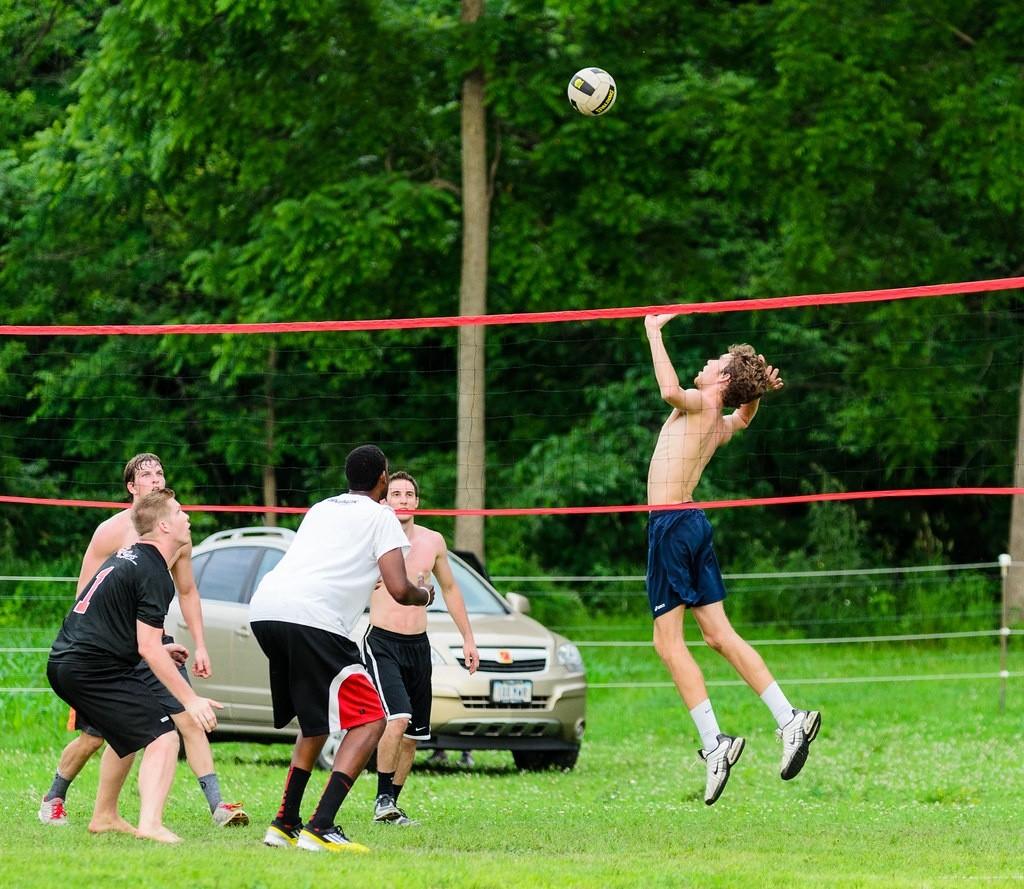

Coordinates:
[567,66,617,117]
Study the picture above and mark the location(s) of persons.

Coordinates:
[38,453,248,827]
[423,749,476,768]
[249,446,436,853]
[46,488,224,843]
[359,470,479,823]
[645,312,822,806]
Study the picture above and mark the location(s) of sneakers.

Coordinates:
[263,819,304,849]
[37,796,68,825]
[688,734,745,806]
[212,801,249,827]
[776,708,821,780]
[372,794,401,821]
[384,807,421,828]
[297,824,371,854]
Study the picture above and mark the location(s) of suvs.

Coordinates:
[162,526,588,775]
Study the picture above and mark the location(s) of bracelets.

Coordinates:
[420,587,430,606]
